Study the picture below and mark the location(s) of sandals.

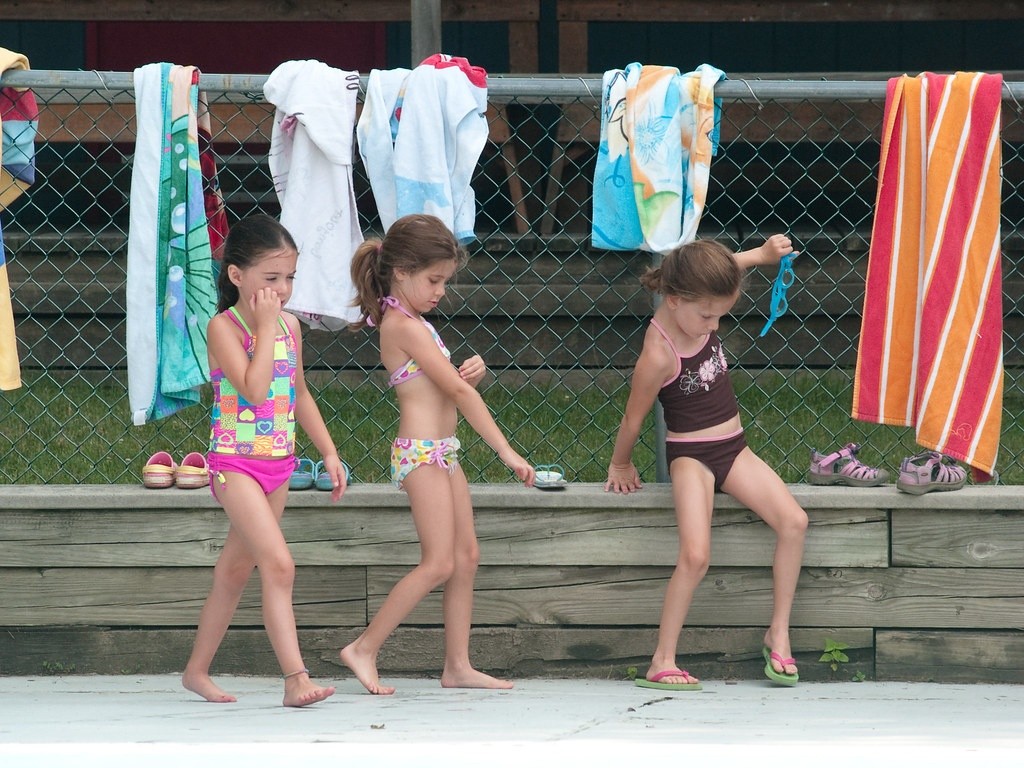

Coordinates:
[808,443,891,486]
[898,451,967,495]
[174,450,210,487]
[142,450,177,488]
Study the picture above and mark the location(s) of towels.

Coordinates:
[125,63,231,426]
[850,69,1004,484]
[0,44,40,392]
[262,59,365,331]
[357,52,491,245]
[591,61,727,256]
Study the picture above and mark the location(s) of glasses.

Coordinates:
[770,268,793,318]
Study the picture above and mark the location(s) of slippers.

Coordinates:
[534,463,567,487]
[290,458,316,489]
[634,670,702,691]
[760,645,800,687]
[315,458,352,490]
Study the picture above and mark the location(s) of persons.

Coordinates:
[602,232,810,691]
[180,215,346,709]
[340,216,535,697]
